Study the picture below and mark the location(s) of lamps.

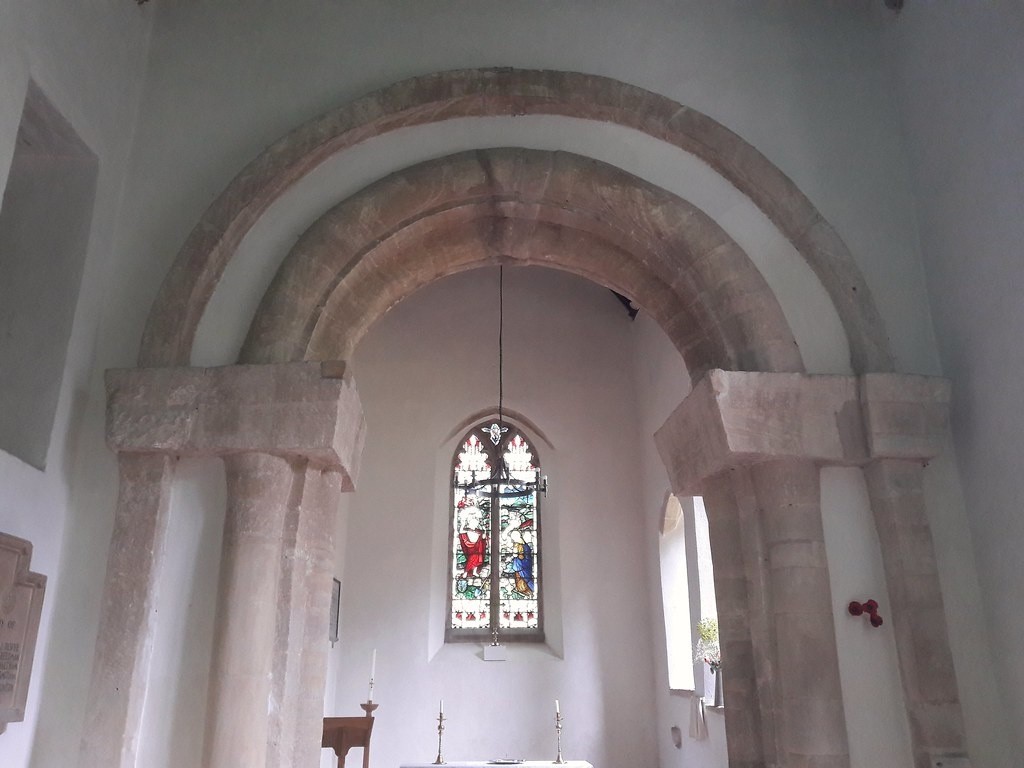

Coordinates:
[452,263,550,503]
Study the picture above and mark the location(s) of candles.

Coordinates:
[370,648,378,680]
[555,698,560,713]
[440,698,444,714]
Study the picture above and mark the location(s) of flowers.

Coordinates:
[692,616,721,673]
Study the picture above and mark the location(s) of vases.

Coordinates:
[714,668,724,706]
